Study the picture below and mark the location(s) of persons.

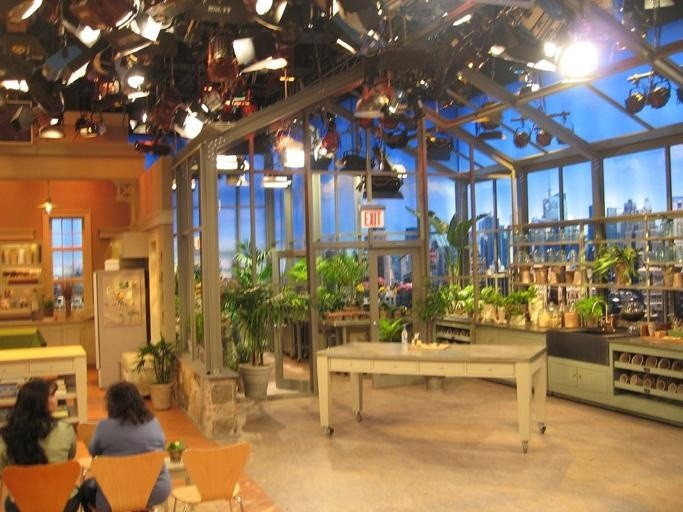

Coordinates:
[0,374,85,511]
[354,275,412,307]
[80,379,172,511]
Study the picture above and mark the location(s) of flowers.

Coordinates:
[166,437,185,450]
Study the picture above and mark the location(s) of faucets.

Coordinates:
[592,299,614,329]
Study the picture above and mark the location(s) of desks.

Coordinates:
[316,338,548,456]
[0,328,47,347]
[0,342,88,424]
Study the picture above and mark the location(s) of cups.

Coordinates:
[3,242,41,266]
[647,321,655,338]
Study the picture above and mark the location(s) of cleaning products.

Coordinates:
[539,301,577,329]
[31,289,40,312]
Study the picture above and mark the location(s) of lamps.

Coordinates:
[35,176,60,215]
[1,0,389,141]
[387,1,630,83]
[378,80,453,162]
[471,85,570,145]
[164,138,409,199]
[626,84,670,114]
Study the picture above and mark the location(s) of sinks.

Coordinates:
[568,329,618,335]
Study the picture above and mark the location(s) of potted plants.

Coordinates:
[435,279,603,329]
[223,238,305,399]
[134,333,177,411]
[316,255,410,321]
[412,276,447,389]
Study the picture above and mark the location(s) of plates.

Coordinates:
[618,352,683,395]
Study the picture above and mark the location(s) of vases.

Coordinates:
[168,449,183,463]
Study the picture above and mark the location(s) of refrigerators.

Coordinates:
[91,268,146,392]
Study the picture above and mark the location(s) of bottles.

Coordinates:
[548,300,577,312]
[401,323,407,344]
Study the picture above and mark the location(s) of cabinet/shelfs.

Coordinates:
[608,341,682,424]
[506,209,682,338]
[434,322,473,344]
[548,357,608,408]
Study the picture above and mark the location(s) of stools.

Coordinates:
[119,351,168,397]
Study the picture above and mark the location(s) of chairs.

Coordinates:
[171,439,253,512]
[0,458,84,511]
[89,448,168,510]
